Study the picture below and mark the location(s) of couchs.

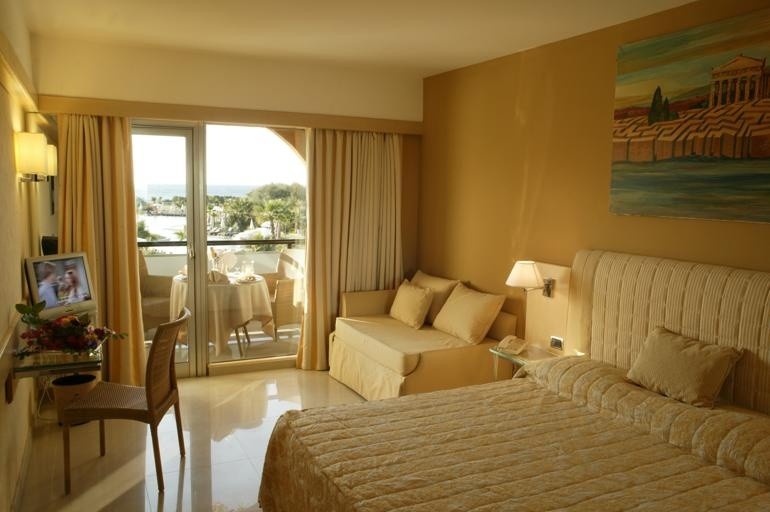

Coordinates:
[328,287,518,402]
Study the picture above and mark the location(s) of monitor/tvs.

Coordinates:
[25,251,97,324]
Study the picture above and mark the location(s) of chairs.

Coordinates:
[137,250,175,333]
[62,306,193,494]
[260,251,305,343]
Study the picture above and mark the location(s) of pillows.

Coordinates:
[625,325,745,409]
[388,277,433,330]
[412,268,459,323]
[430,282,508,346]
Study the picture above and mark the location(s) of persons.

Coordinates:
[36,262,57,310]
[63,268,85,304]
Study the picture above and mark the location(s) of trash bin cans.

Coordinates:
[52,374,98,427]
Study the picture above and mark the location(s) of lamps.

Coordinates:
[505,260,554,299]
[15,130,60,183]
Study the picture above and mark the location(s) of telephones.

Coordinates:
[497,335,528,355]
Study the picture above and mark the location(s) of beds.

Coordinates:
[253,248,770,511]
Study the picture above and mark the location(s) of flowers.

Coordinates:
[12,305,131,360]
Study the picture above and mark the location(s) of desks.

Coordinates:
[488,345,568,380]
[168,274,278,357]
